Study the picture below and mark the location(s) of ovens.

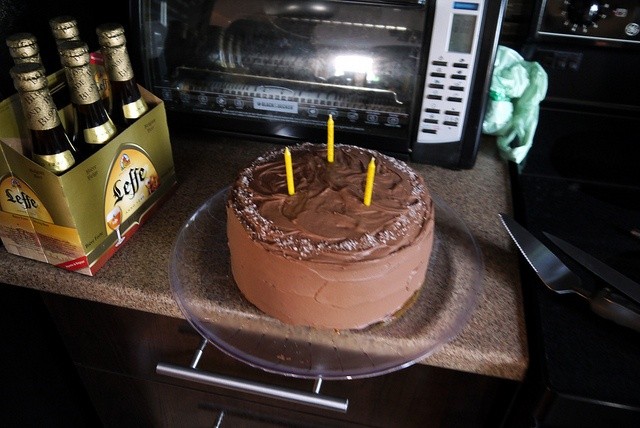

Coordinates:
[0,283,530,427]
[127,0,508,171]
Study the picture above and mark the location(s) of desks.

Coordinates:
[0,100,527,427]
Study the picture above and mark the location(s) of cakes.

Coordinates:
[224,114,435,329]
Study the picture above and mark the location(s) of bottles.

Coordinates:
[49,14,82,47]
[9,62,81,175]
[5,32,42,65]
[95,22,149,130]
[58,39,120,158]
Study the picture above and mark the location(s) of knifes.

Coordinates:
[540,229,640,303]
